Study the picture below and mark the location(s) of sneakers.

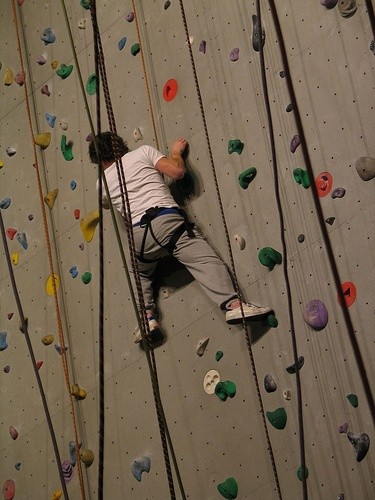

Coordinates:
[133,316,160,343]
[225,302,272,325]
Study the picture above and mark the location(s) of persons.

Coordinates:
[89,132,273,343]
